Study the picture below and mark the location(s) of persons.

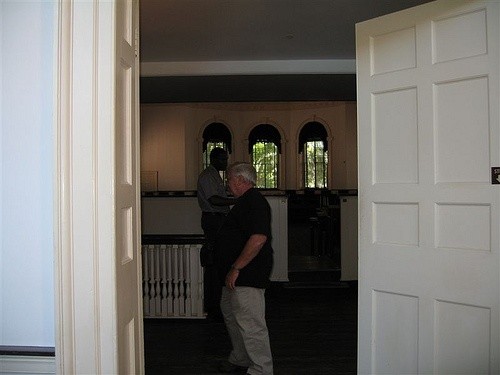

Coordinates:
[218,161,275,375]
[197,147,238,323]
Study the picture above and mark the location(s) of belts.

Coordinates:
[202,212,227,216]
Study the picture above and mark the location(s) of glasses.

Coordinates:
[225,177,231,182]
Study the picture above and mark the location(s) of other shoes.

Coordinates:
[218,361,247,373]
[206,313,224,322]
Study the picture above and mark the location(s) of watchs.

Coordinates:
[231,264,241,271]
